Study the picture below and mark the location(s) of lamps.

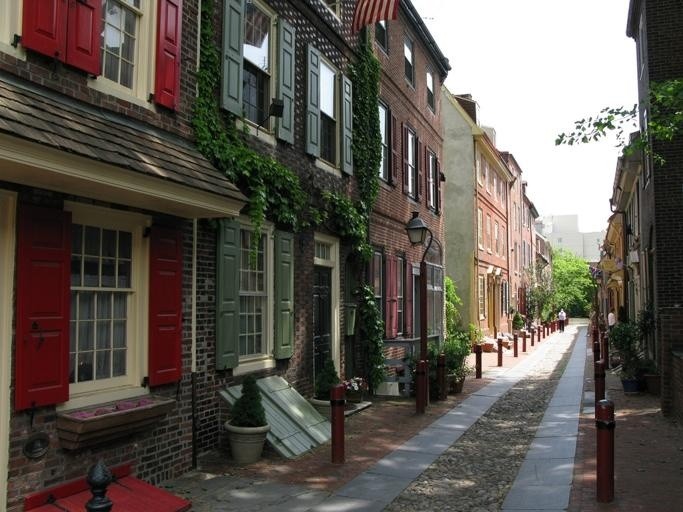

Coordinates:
[257,98,285,130]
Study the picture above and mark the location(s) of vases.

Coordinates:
[347,392,361,403]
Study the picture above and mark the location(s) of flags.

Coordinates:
[590,267,599,277]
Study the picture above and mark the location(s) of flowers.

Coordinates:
[342,376,370,393]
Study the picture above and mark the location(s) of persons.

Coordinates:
[618,305,628,324]
[558,308,566,332]
[607,307,616,333]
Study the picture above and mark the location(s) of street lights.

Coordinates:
[404,210,433,414]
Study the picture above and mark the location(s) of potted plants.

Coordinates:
[224,375,270,464]
[311,359,345,420]
[609,299,661,397]
[445,337,471,393]
[513,311,524,337]
